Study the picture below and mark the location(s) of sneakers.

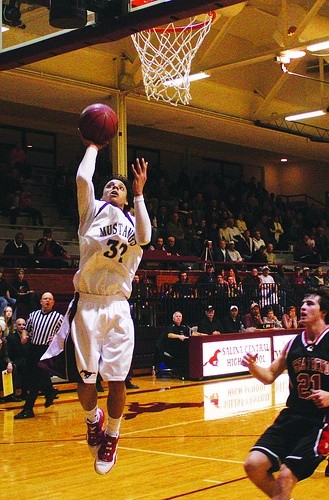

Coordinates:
[87,408,105,457]
[94,432,120,476]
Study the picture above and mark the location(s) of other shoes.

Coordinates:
[96,380,105,392]
[14,410,35,419]
[44,389,58,408]
[126,381,140,389]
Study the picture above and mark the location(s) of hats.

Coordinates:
[229,305,238,312]
[262,265,270,270]
[250,304,258,310]
[302,266,309,271]
[204,304,216,311]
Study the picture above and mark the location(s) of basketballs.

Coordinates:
[78,103,117,144]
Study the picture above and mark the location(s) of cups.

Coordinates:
[192,326,197,332]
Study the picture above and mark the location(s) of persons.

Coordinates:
[245,303,264,329]
[223,305,246,334]
[37,128,152,476]
[13,292,65,419]
[0,142,43,225]
[143,166,329,308]
[0,266,37,402]
[240,287,329,500]
[281,306,298,329]
[199,305,223,335]
[168,311,208,380]
[262,308,283,328]
[5,228,71,268]
[53,165,76,216]
